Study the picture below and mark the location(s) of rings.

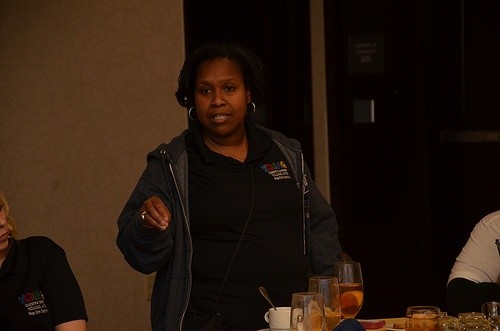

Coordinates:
[141,211,147,219]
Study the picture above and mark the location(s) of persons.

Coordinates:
[442,210,500,319]
[116,39,339,331]
[0,193,88,331]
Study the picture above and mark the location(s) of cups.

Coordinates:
[332,261,364,319]
[290,292,324,331]
[264,307,303,331]
[405,306,443,331]
[308,275,341,330]
[440,301,500,331]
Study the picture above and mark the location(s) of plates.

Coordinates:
[382,323,405,331]
